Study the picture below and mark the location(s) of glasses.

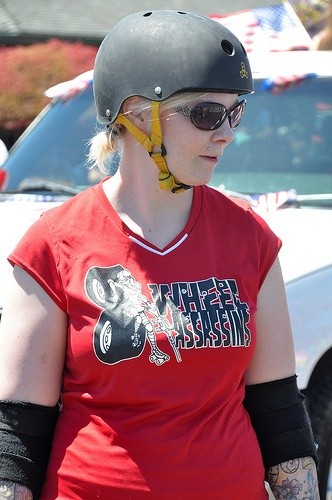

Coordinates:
[174,99,246,131]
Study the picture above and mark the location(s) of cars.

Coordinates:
[0,49,331,500]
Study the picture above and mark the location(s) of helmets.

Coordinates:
[93,9,254,126]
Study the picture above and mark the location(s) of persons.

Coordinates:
[0,8,323,500]
